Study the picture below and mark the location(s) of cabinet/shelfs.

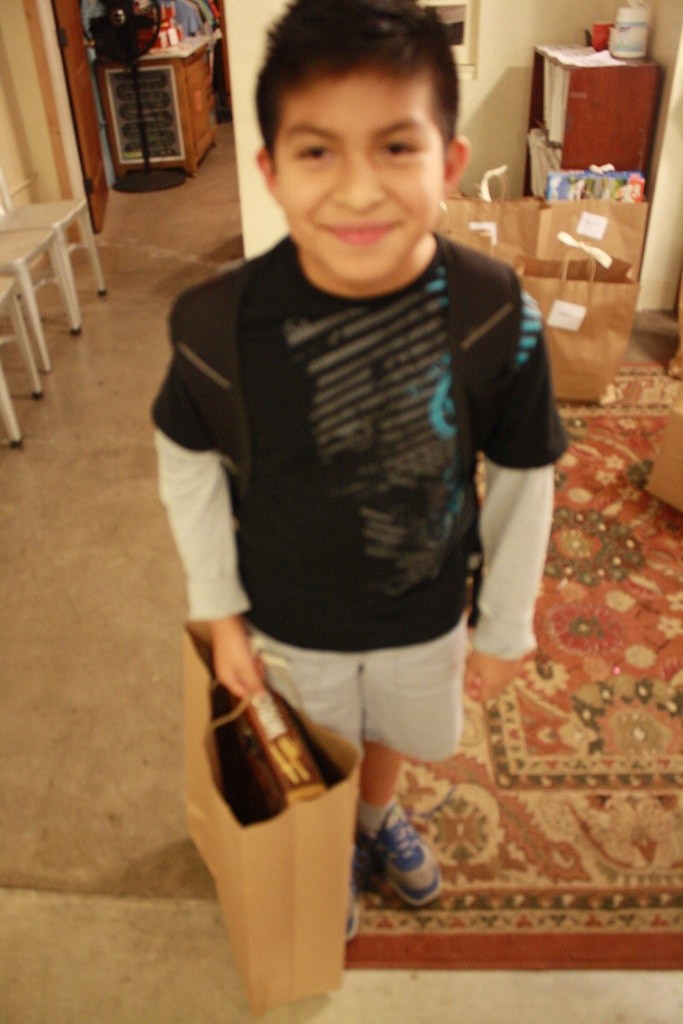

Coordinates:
[91,34,219,180]
[522,42,662,202]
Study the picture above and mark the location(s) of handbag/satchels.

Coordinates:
[176,621,362,1017]
[645,385,683,513]
[436,163,648,402]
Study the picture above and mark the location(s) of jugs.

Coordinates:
[611,0,651,59]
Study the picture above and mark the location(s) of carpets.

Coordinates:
[262,360,683,970]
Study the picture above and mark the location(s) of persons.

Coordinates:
[152,1,565,943]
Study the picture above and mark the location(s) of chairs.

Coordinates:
[0,167,107,449]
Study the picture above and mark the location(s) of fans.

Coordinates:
[79,0,187,195]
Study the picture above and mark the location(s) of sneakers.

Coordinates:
[358,803,443,905]
[342,846,362,942]
